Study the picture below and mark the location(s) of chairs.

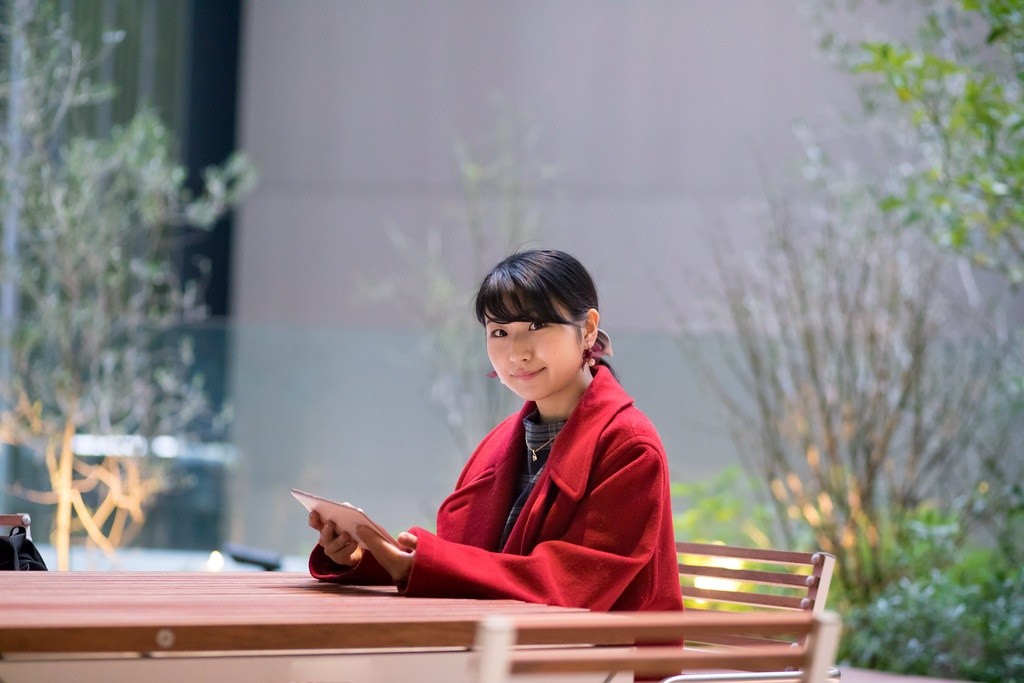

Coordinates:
[460,612,841,682]
[660,541,836,683]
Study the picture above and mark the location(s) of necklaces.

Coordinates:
[525,434,555,462]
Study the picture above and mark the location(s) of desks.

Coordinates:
[0,572,595,661]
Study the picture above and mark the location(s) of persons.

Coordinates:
[310,248,684,682]
